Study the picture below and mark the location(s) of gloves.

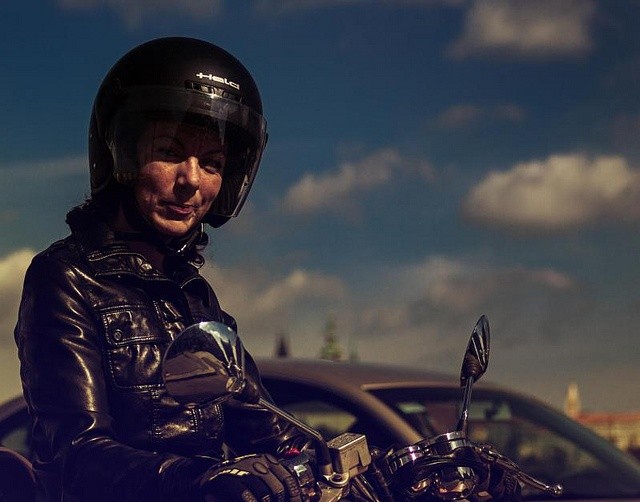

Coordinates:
[157,452,304,502]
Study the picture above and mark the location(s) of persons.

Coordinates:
[12,36,315,497]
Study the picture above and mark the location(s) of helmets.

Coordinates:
[88,36,269,229]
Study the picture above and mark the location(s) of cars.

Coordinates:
[0,358,640,502]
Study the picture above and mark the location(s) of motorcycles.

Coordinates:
[0,316,563,502]
